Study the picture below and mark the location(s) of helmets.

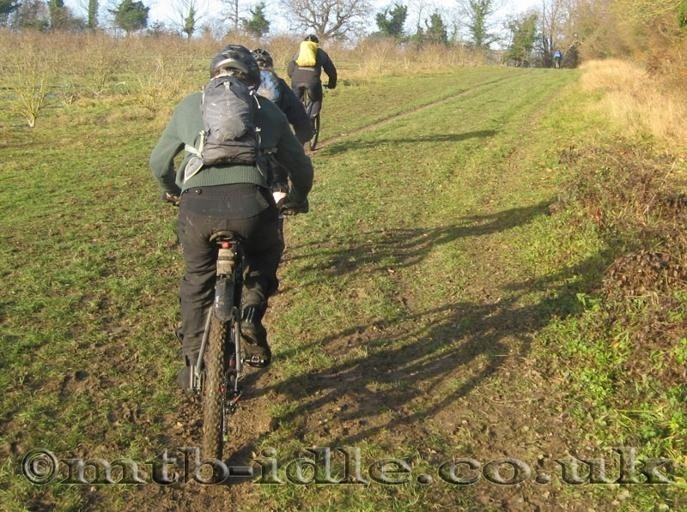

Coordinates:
[210,35,319,91]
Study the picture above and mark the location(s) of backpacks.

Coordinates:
[256,71,281,103]
[200,76,262,166]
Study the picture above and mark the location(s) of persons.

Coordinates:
[149,44,314,396]
[286,32,337,117]
[554,49,562,63]
[253,47,314,193]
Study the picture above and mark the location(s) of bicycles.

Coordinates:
[554,59,560,69]
[155,190,308,481]
[297,83,331,152]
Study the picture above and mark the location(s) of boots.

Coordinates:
[175,326,202,403]
[241,305,271,368]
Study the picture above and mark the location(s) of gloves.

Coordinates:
[278,197,308,215]
